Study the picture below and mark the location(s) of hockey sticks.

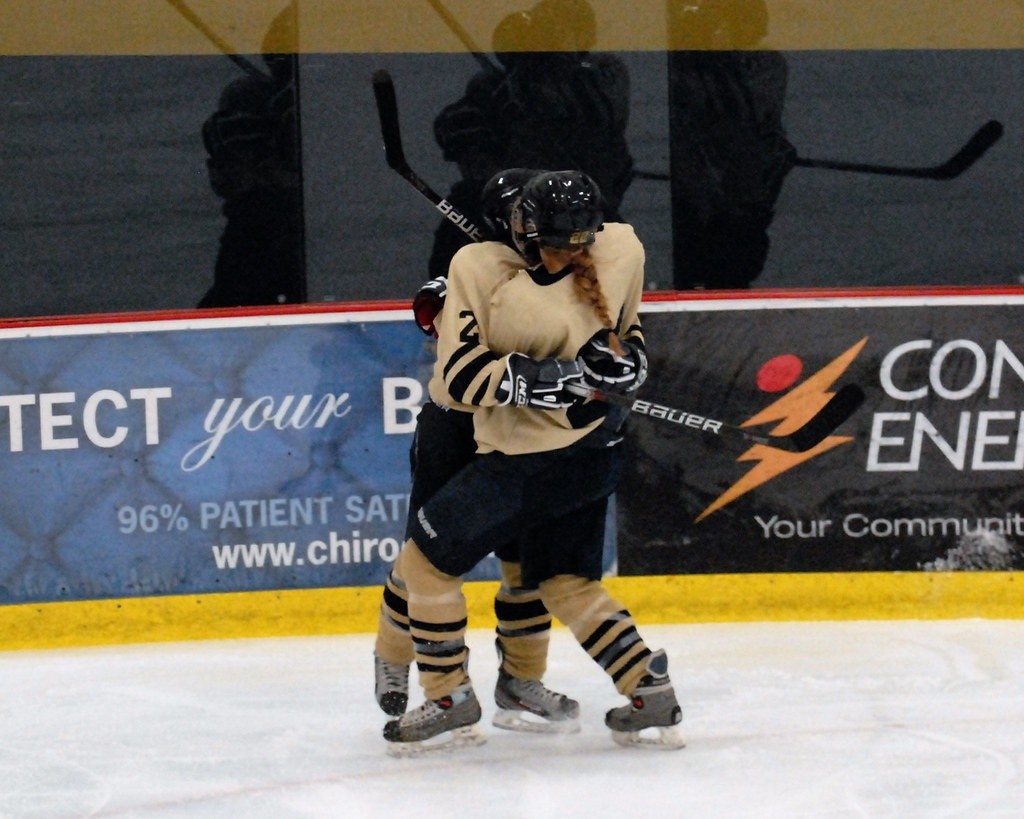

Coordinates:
[425,0,496,73]
[630,166,671,184]
[169,0,266,78]
[372,66,865,452]
[796,118,1006,181]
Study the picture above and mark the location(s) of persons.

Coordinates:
[192,3,795,305]
[385,173,683,742]
[373,171,647,726]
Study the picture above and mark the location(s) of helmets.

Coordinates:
[480,168,549,243]
[532,0,597,59]
[511,169,605,256]
[667,0,767,57]
[492,12,534,69]
[259,9,297,82]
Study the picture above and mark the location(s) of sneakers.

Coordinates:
[373,655,410,721]
[382,644,489,759]
[492,636,579,734]
[605,650,687,751]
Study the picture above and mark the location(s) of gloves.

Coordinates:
[493,351,584,411]
[576,328,648,394]
[412,275,450,336]
